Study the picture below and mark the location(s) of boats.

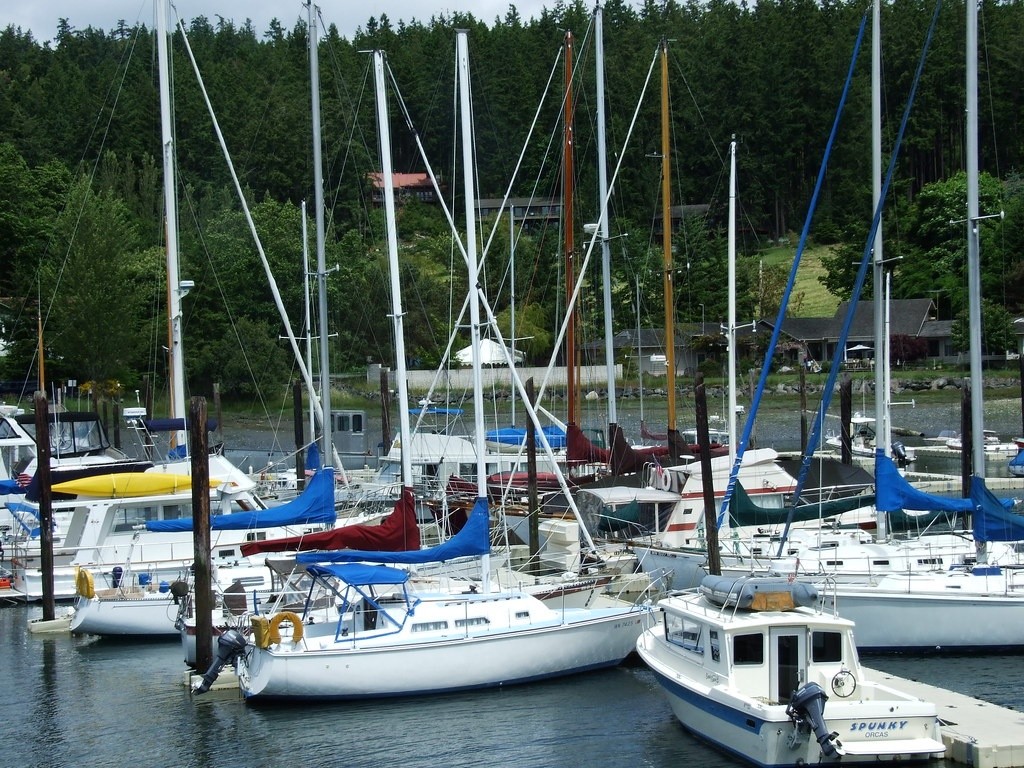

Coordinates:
[635,571,948,765]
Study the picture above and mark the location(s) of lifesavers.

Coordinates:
[78,570,95,599]
[270,612,303,644]
[661,470,671,492]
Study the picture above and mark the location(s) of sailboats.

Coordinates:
[0,1,1024,704]
